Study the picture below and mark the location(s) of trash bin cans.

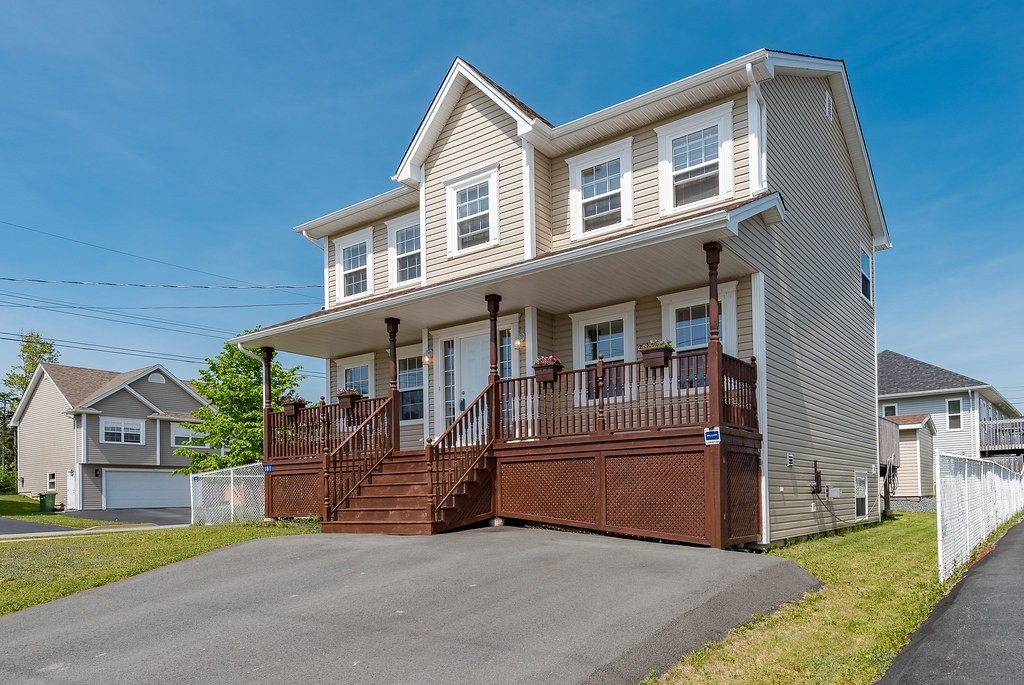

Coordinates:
[38,491,58,513]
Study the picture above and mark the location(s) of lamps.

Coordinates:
[423,349,432,365]
[514,332,525,348]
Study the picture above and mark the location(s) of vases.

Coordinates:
[282,402,307,416]
[642,347,669,367]
[532,364,565,382]
[334,394,363,408]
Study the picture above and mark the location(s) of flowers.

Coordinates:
[336,386,358,395]
[636,338,672,350]
[282,395,305,403]
[535,353,561,365]
